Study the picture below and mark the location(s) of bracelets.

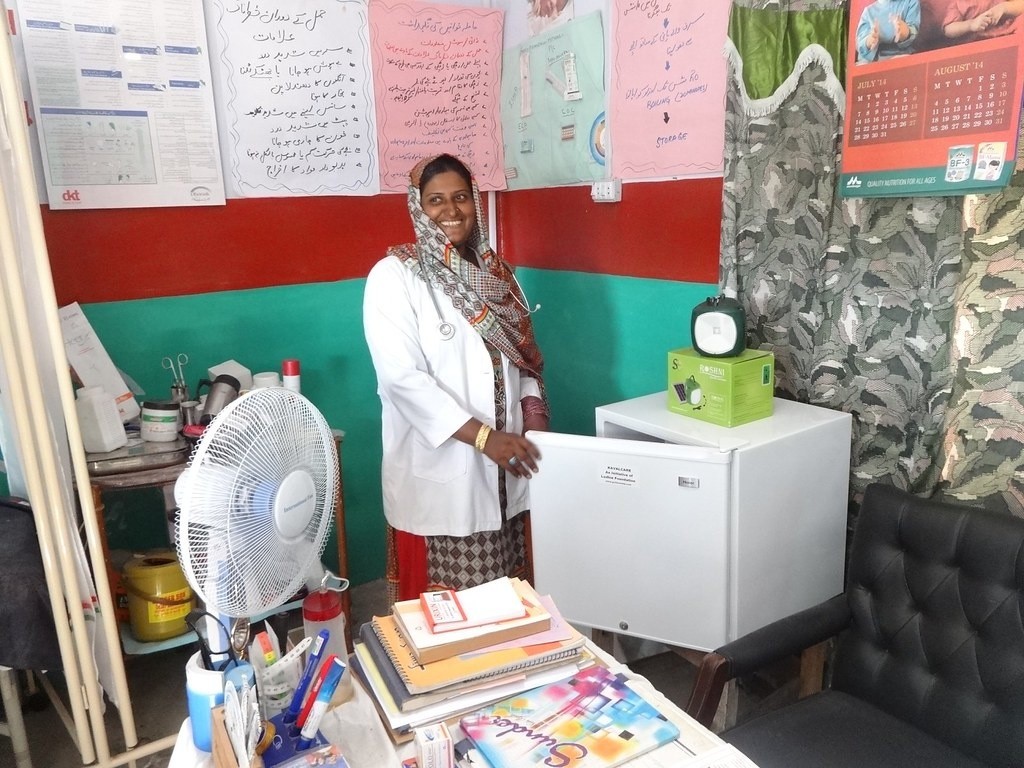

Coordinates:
[475,424,492,454]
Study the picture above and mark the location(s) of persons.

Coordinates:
[361,152,551,614]
[854,0,1024,66]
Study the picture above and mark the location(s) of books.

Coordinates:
[349,573,597,747]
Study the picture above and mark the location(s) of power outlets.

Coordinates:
[590,178,622,203]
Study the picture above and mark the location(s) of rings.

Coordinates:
[508,456,517,466]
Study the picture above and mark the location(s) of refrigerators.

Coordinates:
[525,394,852,649]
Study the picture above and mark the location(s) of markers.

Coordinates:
[281,630,331,721]
[293,654,345,751]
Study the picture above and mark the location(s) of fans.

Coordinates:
[169,382,340,743]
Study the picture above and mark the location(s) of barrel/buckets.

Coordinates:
[122,554,199,642]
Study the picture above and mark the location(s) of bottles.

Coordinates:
[252,370,281,393]
[302,572,354,708]
[140,399,179,442]
[75,385,129,454]
[282,359,301,396]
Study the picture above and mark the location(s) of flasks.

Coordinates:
[196,374,241,427]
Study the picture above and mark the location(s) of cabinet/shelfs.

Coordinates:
[521,388,851,652]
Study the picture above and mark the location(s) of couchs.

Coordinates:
[688,482,1024,768]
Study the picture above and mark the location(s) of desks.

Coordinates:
[164,633,762,768]
[82,425,355,655]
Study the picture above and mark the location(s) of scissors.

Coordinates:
[161,353,189,388]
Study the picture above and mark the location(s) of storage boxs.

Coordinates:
[207,359,252,389]
[59,301,141,423]
[664,346,780,429]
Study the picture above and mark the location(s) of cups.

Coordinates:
[182,401,199,425]
[171,385,189,402]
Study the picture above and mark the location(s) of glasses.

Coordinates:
[184,608,239,671]
